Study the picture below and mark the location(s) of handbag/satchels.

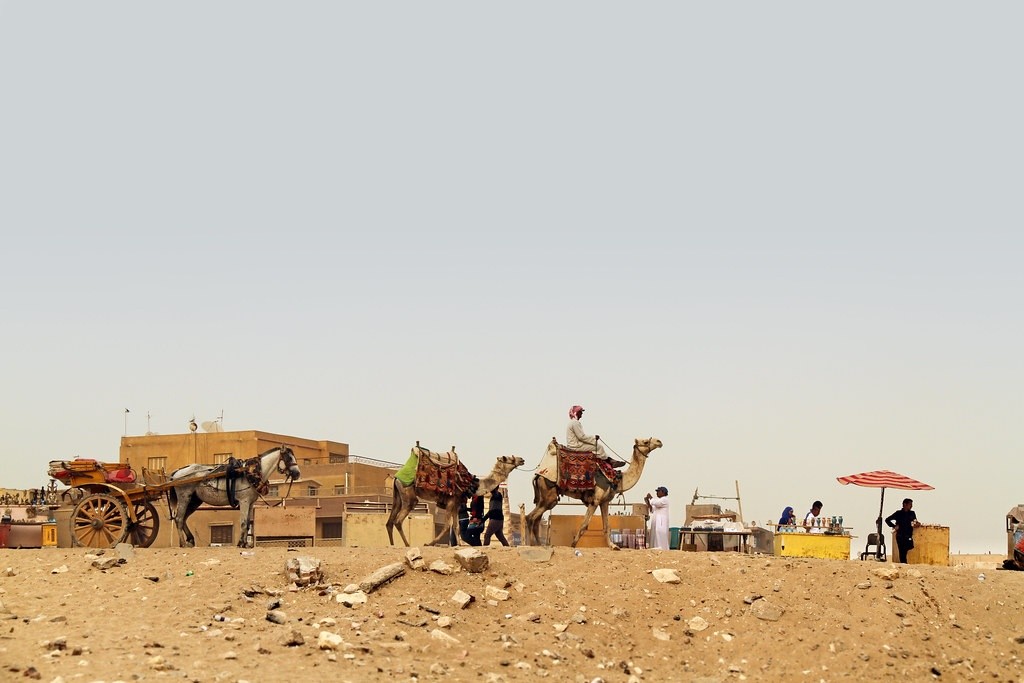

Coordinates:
[467,517,485,533]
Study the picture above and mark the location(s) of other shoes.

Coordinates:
[612,461,626,468]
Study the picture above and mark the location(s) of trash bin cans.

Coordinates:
[669,527,682,549]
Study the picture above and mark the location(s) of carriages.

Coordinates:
[47,442,302,552]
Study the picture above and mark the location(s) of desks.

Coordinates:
[547,514,646,548]
[678,530,752,553]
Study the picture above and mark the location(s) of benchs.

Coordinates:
[69,459,134,487]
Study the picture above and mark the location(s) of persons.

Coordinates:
[644,486,670,550]
[777,507,797,532]
[566,405,626,468]
[885,499,921,564]
[803,501,823,533]
[451,485,509,546]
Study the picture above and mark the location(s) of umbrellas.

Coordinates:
[836,470,935,560]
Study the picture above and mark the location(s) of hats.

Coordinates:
[656,487,668,493]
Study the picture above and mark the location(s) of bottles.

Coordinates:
[214,615,230,621]
[978,573,985,581]
[575,549,584,558]
[789,516,843,527]
[239,551,255,557]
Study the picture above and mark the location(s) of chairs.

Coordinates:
[861,533,886,562]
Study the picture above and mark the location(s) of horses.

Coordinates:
[166,441,301,550]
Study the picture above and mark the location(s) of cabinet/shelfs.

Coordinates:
[773,523,857,558]
[892,525,950,566]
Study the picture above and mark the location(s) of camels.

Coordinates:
[525,436,663,552]
[385,438,525,547]
